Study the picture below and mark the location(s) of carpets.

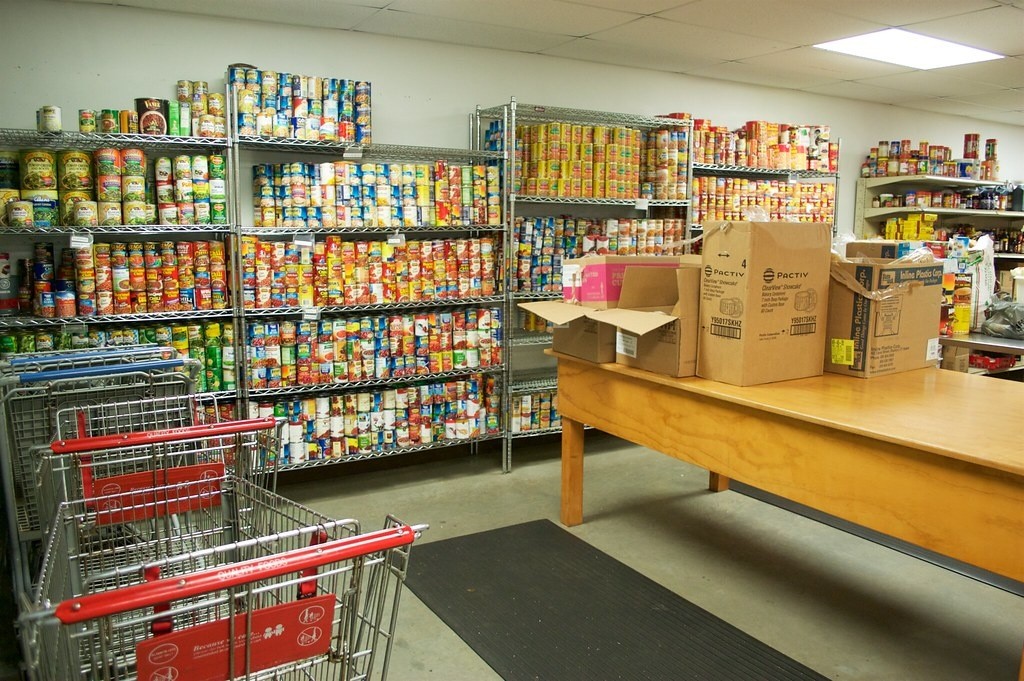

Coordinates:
[383,519,835,681]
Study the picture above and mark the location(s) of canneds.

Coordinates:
[484,116,838,434]
[0,65,499,471]
[859,133,1011,211]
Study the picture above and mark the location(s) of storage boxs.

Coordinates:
[516,220,943,386]
[941,346,969,372]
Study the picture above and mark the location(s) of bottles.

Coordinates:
[991,228,1023,254]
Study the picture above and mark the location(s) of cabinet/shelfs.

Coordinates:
[855,175,1024,259]
[478,97,695,473]
[689,160,840,255]
[1,84,507,515]
[542,348,1024,681]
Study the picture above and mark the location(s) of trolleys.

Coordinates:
[0,343,429,680]
